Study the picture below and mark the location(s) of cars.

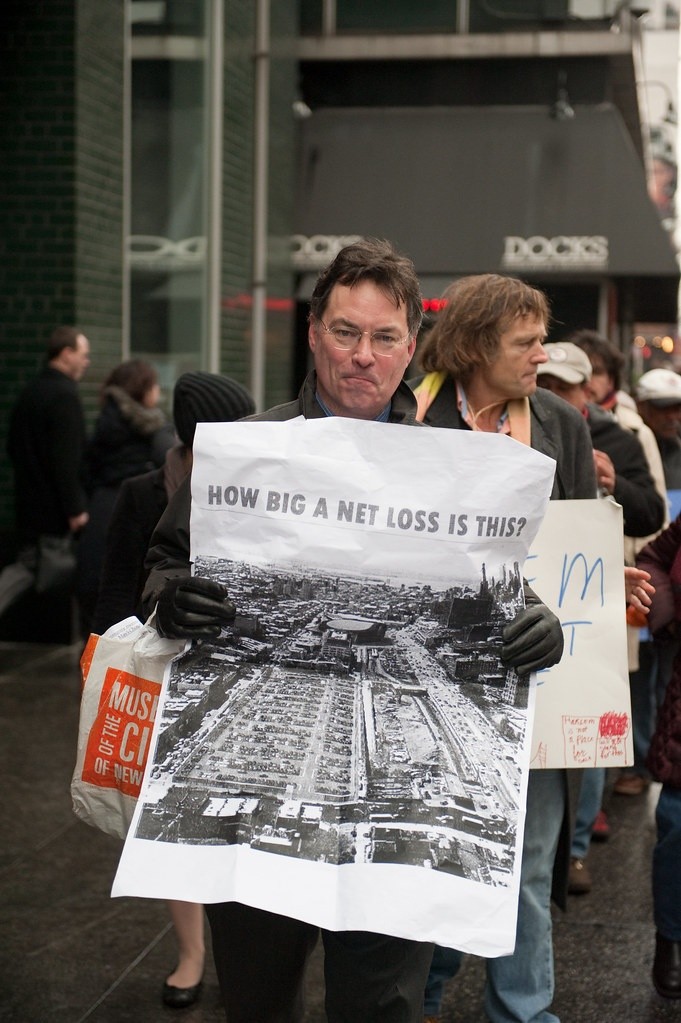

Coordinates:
[133,557,522,887]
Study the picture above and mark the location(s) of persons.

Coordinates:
[141,236,561,1023]
[79,356,176,643]
[536,341,666,893]
[422,275,654,1023]
[613,365,680,793]
[565,330,664,842]
[0,327,91,669]
[634,512,679,1000]
[92,371,256,1007]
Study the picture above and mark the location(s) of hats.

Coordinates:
[535,341,593,384]
[636,367,681,408]
[175,372,256,445]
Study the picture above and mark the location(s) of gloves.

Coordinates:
[500,585,563,676]
[157,577,236,639]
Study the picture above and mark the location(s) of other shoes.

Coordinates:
[565,856,595,894]
[615,775,654,795]
[166,947,208,1006]
[651,933,681,997]
[593,811,611,838]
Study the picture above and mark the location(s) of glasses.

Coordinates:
[315,317,415,356]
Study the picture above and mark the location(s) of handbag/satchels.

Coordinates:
[40,526,83,593]
[71,601,187,841]
